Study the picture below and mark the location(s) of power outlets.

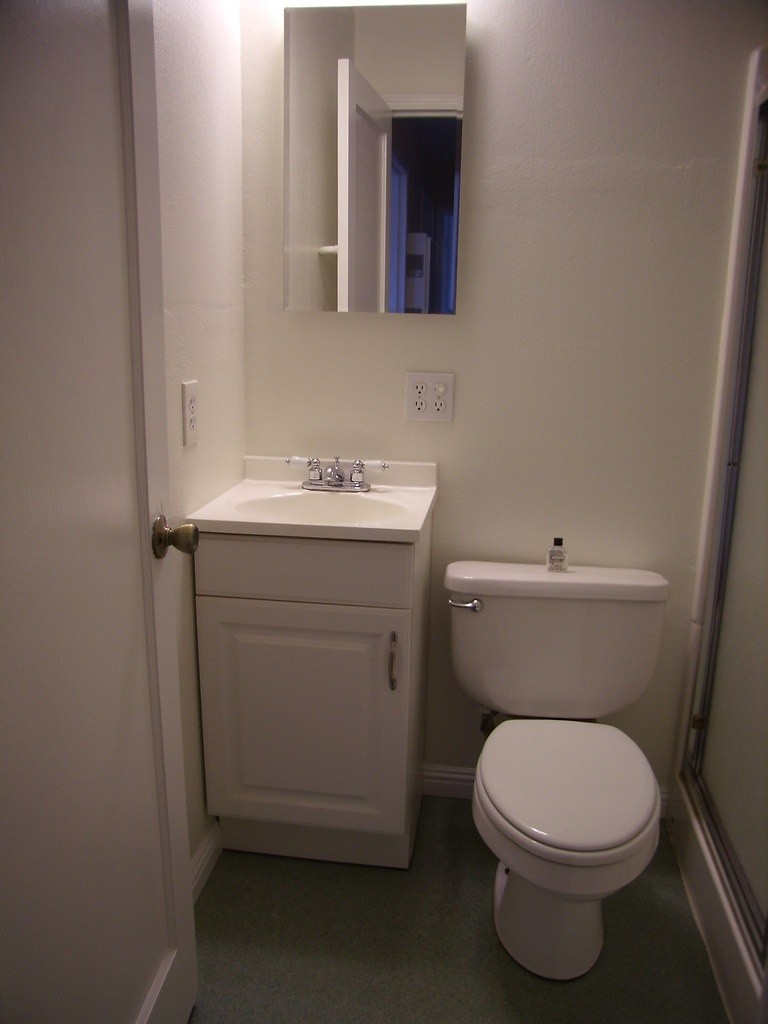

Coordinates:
[404,371,456,425]
[179,378,200,450]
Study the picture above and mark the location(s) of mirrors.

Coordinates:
[283,4,467,315]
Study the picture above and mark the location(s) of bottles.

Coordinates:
[547,538,567,573]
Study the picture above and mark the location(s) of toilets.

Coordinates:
[442,560,670,979]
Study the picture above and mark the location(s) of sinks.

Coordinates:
[236,493,410,524]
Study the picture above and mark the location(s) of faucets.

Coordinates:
[323,456,345,486]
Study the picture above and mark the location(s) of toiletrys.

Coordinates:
[546,536,569,574]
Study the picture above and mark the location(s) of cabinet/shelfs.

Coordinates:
[404,232,431,315]
[186,519,421,869]
